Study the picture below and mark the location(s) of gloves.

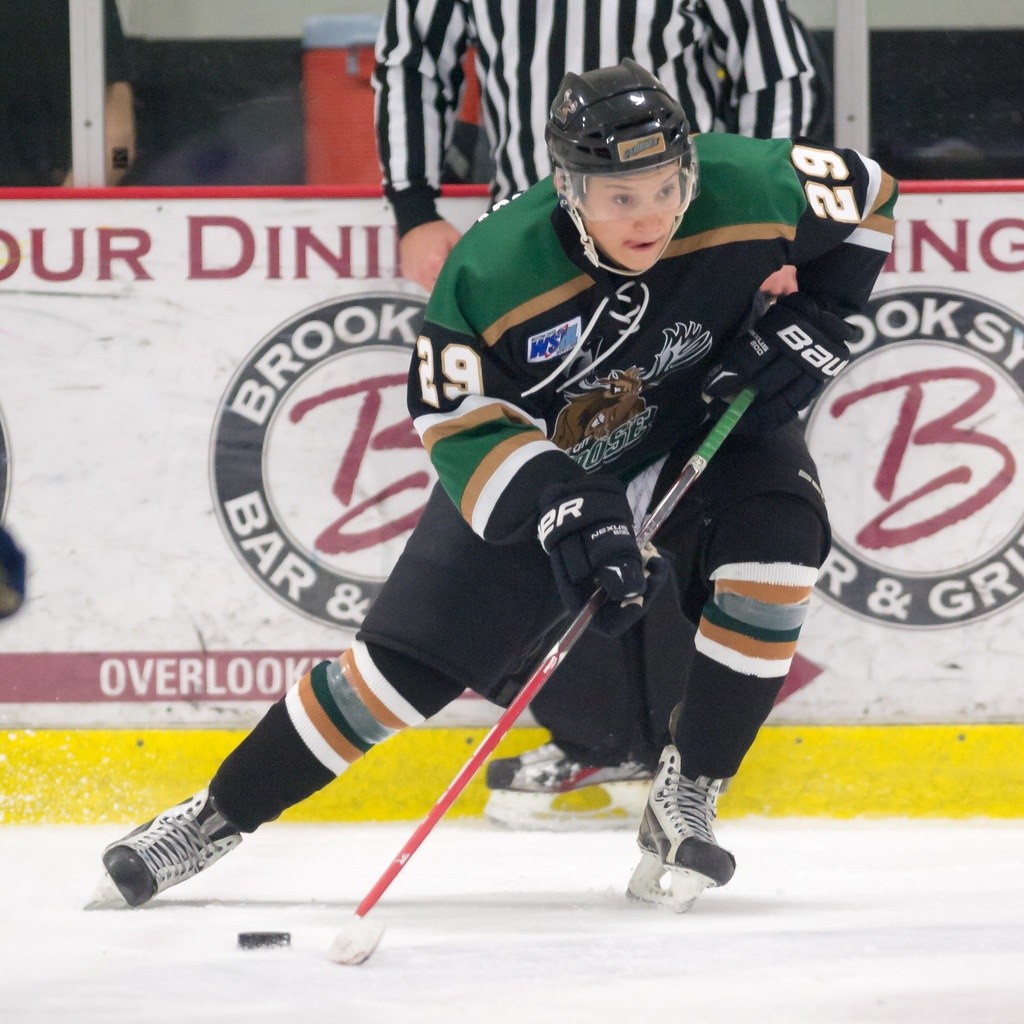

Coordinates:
[703,295,851,431]
[534,472,672,639]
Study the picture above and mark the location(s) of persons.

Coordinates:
[92,57,898,914]
[0,0,136,187]
[371,0,816,834]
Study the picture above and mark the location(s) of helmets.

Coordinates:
[545,56,694,175]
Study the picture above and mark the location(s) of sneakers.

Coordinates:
[99,785,243,906]
[626,746,736,913]
[483,737,655,833]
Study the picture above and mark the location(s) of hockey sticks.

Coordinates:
[325,387,758,965]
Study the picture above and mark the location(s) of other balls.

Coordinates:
[236,930,295,954]
